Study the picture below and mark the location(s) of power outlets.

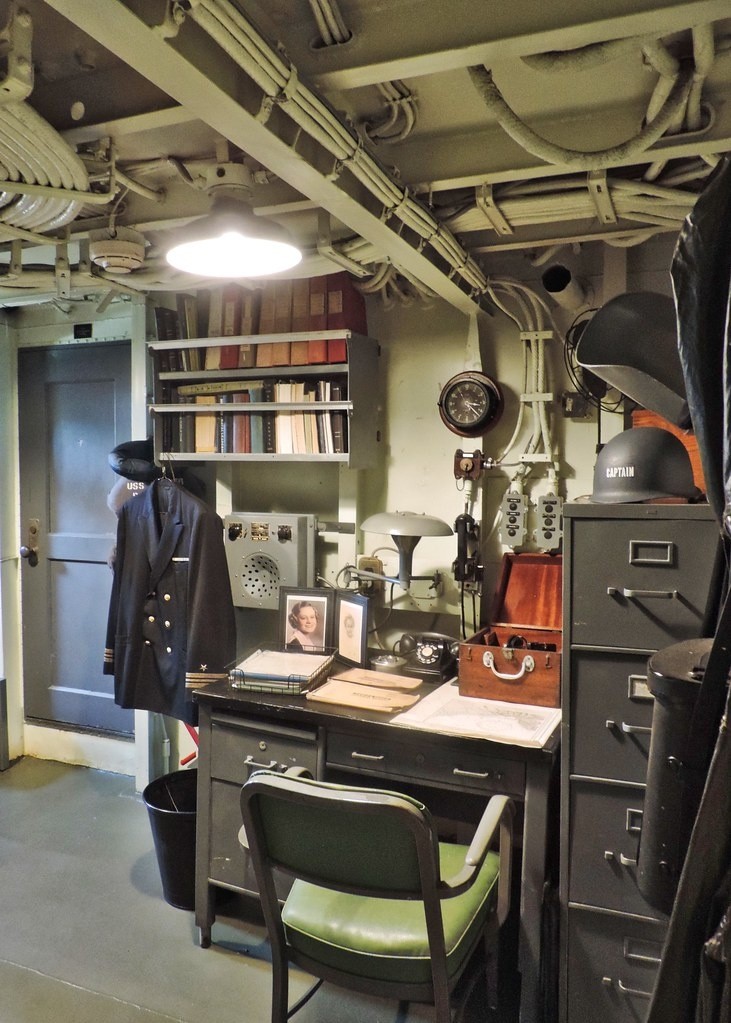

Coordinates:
[357,556,385,593]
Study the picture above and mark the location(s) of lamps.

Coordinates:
[162,185,303,278]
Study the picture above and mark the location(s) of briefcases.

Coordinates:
[458,551,563,708]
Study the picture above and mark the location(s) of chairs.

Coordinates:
[239,770,514,1023]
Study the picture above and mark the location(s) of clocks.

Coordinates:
[436,370,502,436]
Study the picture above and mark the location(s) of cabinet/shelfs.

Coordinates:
[146,328,380,470]
[559,500,719,1023]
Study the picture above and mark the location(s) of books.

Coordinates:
[144,277,364,457]
[229,648,335,694]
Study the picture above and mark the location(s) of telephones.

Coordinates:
[451,512,475,581]
[399,631,459,685]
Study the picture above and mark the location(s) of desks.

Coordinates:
[192,678,562,1023]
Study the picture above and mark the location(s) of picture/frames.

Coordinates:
[278,584,333,657]
[334,587,369,666]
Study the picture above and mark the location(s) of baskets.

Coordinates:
[224,640,339,696]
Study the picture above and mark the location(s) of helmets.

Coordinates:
[593,427,703,504]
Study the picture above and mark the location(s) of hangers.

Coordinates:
[156,464,172,483]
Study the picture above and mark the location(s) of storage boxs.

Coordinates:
[455,551,562,707]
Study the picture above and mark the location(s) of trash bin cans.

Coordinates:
[141,768,239,912]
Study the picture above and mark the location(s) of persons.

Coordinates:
[339,612,363,661]
[288,599,324,653]
